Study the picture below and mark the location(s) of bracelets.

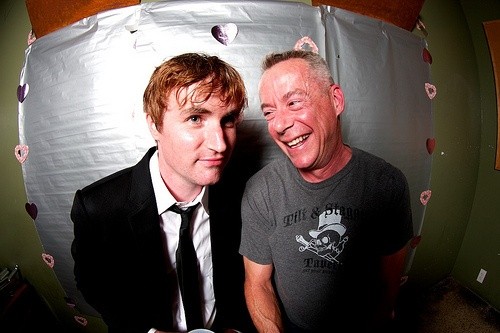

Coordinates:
[384,304,396,311]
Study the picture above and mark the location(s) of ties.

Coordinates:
[168,202,206,332]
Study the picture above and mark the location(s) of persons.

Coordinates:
[70,52,259,333]
[238,49,413,333]
